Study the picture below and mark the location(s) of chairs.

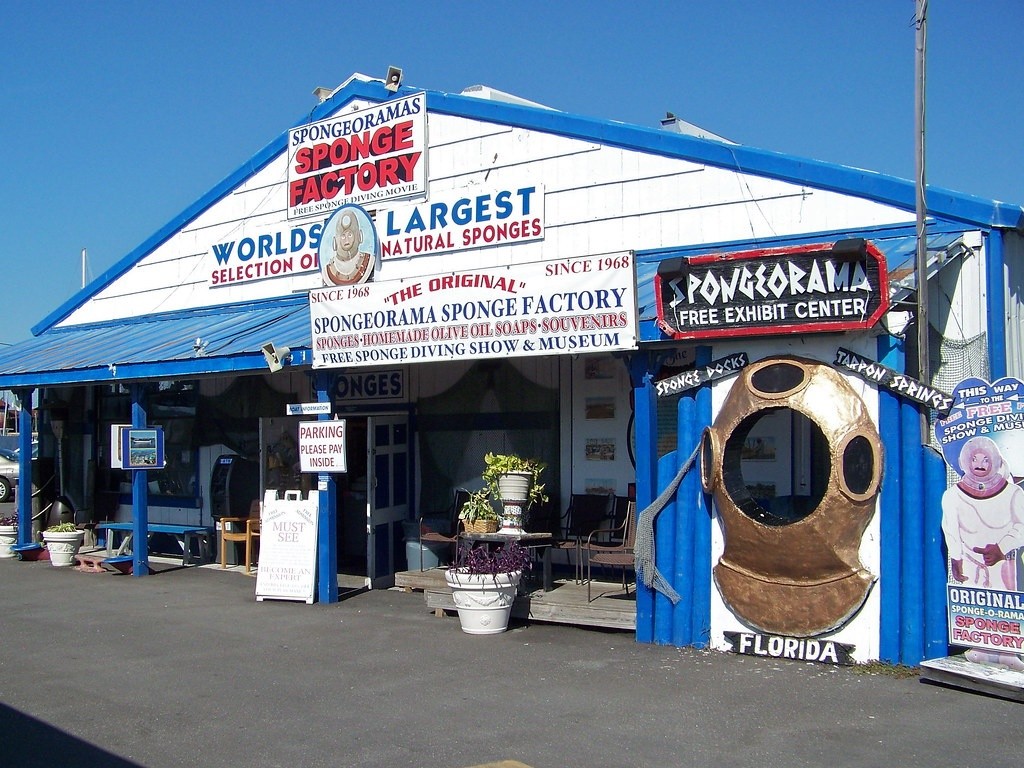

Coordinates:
[536,493,611,586]
[418,489,491,572]
[586,500,637,603]
[219,500,262,573]
[580,494,637,583]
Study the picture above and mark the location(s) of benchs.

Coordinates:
[95,520,215,567]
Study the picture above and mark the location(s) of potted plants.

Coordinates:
[42,522,86,567]
[457,486,500,534]
[445,542,531,635]
[0,513,19,558]
[481,451,550,509]
